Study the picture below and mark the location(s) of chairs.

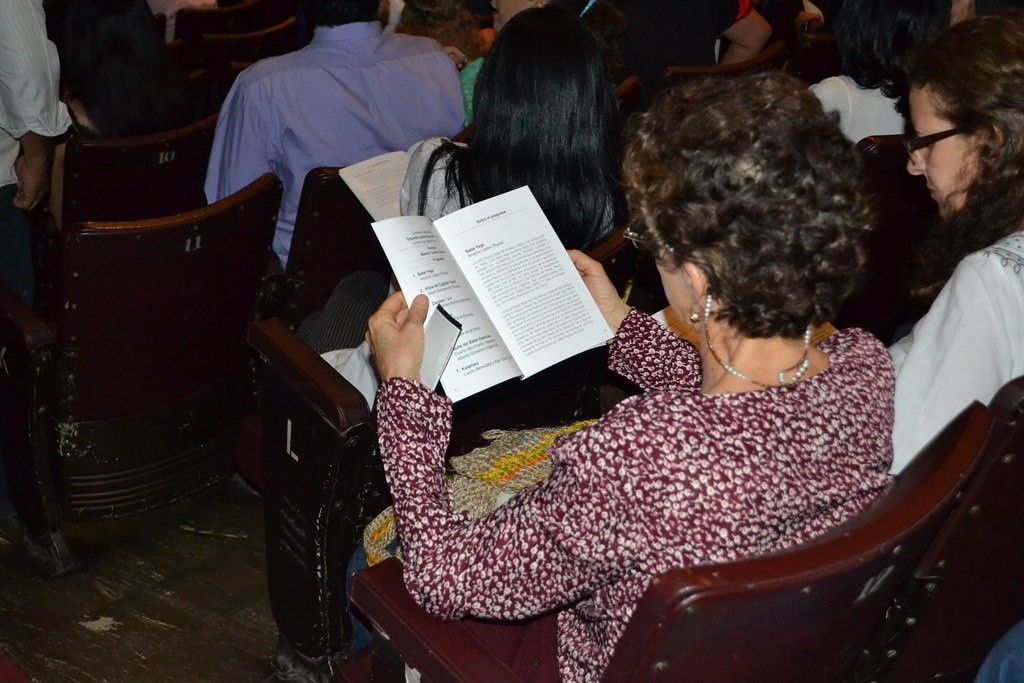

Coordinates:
[0,1,1024,683]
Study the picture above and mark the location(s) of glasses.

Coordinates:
[899,124,959,167]
[620,217,708,266]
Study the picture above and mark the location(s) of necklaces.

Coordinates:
[702,294,819,390]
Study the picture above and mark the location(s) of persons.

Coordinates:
[555,0,777,109]
[393,1,493,127]
[794,0,984,149]
[348,71,898,682]
[395,9,622,258]
[880,12,1024,476]
[2,0,74,264]
[202,0,467,305]
